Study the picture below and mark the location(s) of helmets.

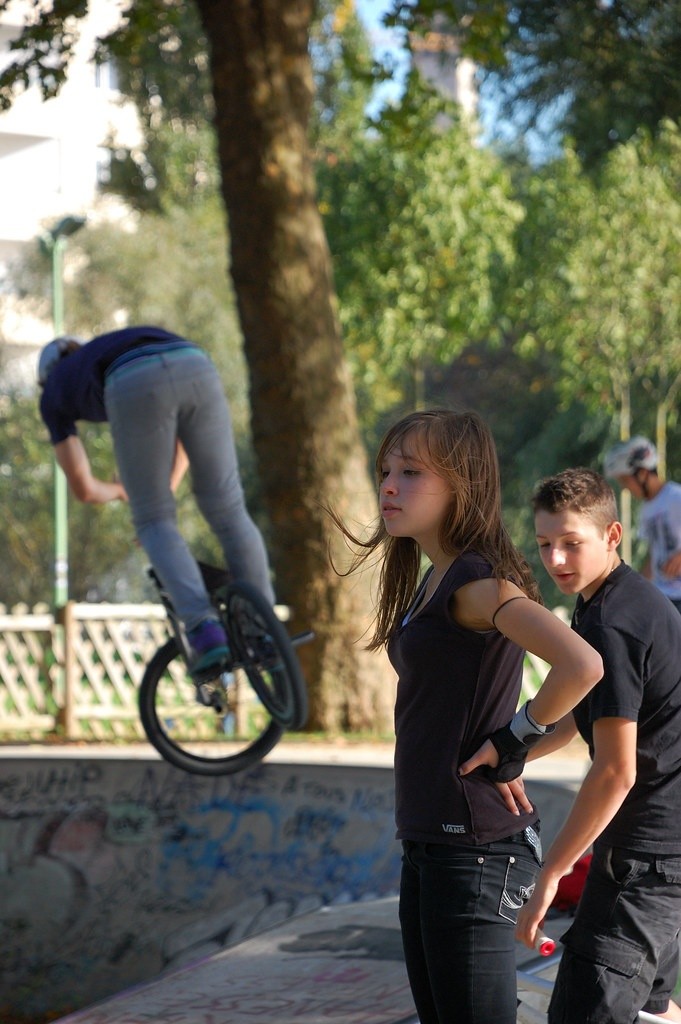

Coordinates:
[603,436,659,477]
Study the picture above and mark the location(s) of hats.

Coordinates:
[36,339,76,381]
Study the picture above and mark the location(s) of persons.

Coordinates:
[322,404,609,1023]
[512,466,680,1023]
[604,434,681,614]
[37,323,279,680]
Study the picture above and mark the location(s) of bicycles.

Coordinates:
[135,551,314,779]
